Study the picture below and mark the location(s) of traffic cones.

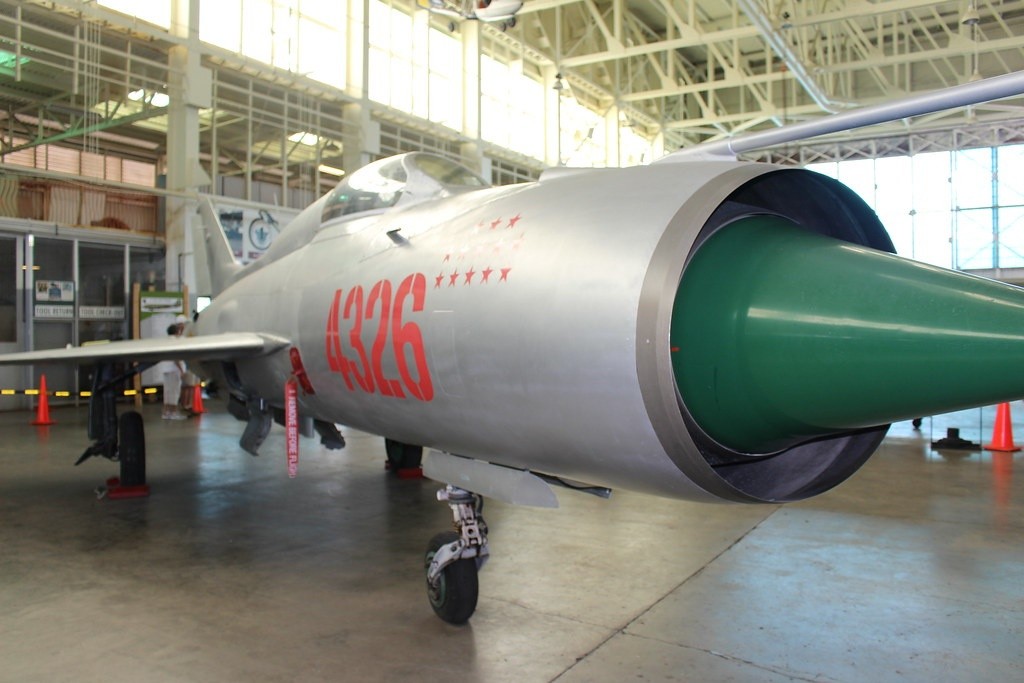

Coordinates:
[30,374,54,425]
[983,403,1022,451]
[192,383,207,413]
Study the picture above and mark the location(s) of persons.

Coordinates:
[159,313,202,423]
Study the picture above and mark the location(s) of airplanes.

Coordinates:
[0,156,1024,624]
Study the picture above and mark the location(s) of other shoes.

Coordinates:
[162,412,187,421]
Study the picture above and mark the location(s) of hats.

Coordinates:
[175,314,190,325]
